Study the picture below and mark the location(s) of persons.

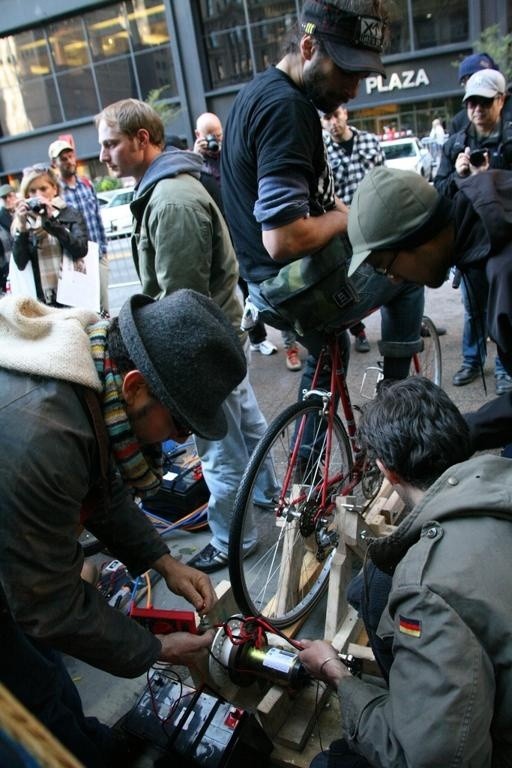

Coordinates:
[0,98,281,574]
[299,373,512,767]
[1,288,248,767]
[221,0,512,502]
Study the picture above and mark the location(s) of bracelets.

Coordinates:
[320,656,339,674]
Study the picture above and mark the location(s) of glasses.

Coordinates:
[373,249,400,276]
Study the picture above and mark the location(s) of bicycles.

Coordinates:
[224,294,450,637]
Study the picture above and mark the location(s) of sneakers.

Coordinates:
[353,335,371,353]
[249,339,279,356]
[186,542,259,575]
[285,347,302,371]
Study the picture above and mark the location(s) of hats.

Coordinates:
[20,163,60,198]
[48,140,75,159]
[457,52,495,85]
[461,68,506,104]
[347,162,442,278]
[0,184,12,197]
[117,286,249,440]
[299,0,388,79]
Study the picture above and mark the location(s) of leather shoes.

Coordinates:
[423,327,447,337]
[452,364,482,386]
[495,373,512,394]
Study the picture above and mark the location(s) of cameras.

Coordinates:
[26,197,42,212]
[206,134,219,152]
[470,148,489,167]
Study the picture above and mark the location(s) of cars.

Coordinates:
[376,129,435,182]
[101,187,136,240]
[96,195,110,209]
